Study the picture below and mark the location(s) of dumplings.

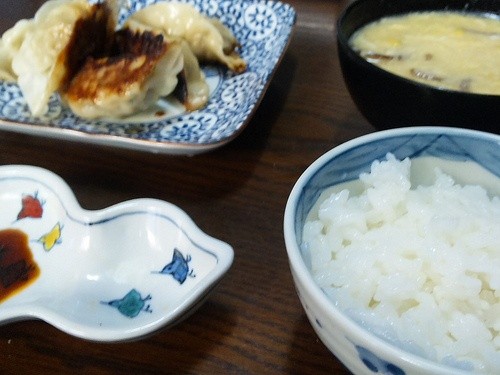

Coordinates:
[0,0,246,112]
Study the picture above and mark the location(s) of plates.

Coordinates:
[0,0,297,156]
[0,163,234,343]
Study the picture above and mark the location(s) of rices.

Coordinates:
[300,151,500,375]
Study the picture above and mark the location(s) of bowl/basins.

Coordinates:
[282,126,500,375]
[334,0,500,134]
